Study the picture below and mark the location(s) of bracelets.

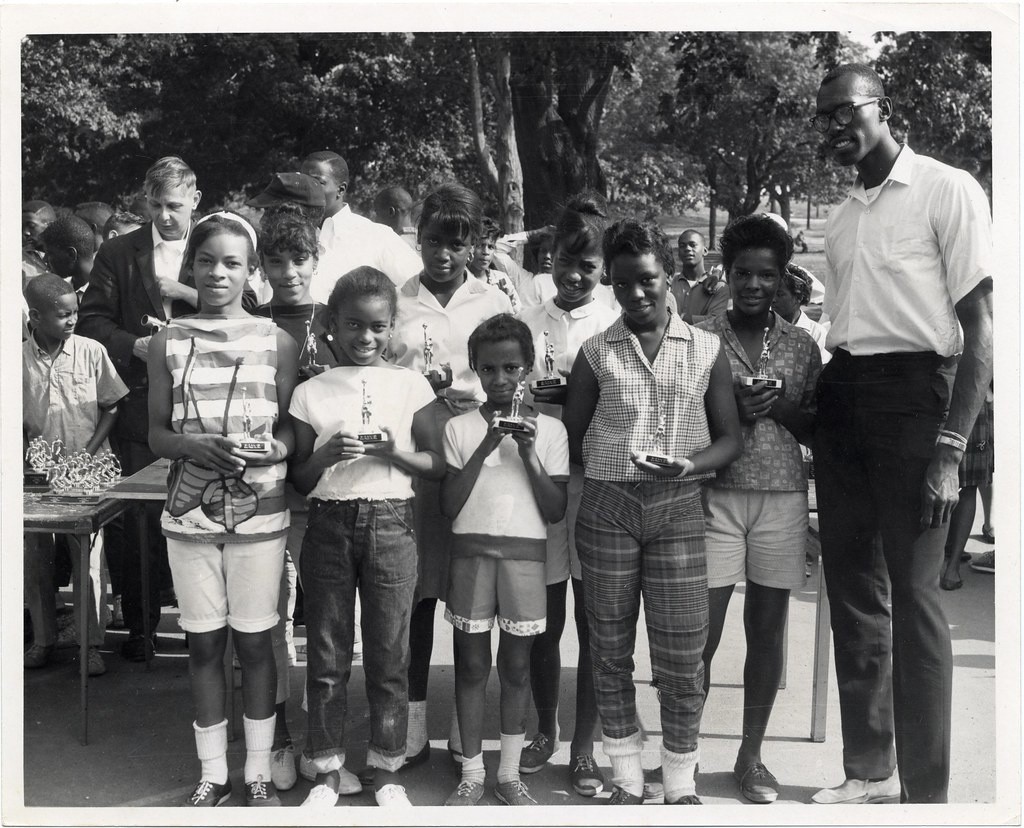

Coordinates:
[934,430,967,451]
[674,458,690,478]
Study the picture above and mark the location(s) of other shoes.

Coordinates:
[358,738,431,785]
[126,630,155,665]
[105,605,112,625]
[113,594,124,621]
[24,643,54,669]
[641,762,699,799]
[811,767,903,804]
[80,647,105,674]
[448,737,463,776]
[55,592,64,609]
[663,792,702,805]
[607,786,645,805]
[942,520,995,590]
[733,759,779,802]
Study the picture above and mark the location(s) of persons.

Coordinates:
[813,63,993,804]
[441,313,570,806]
[770,264,832,368]
[288,267,447,807]
[516,199,623,794]
[247,150,423,376]
[23,273,129,677]
[386,183,514,764]
[645,214,822,802]
[944,380,995,591]
[148,209,298,807]
[22,199,147,340]
[562,216,743,805]
[670,229,728,324]
[24,434,122,490]
[73,157,258,661]
[467,215,558,309]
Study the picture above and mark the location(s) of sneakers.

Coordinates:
[299,752,362,794]
[245,774,281,806]
[493,779,539,805]
[375,784,413,807]
[300,784,341,808]
[517,733,560,774]
[445,781,486,806]
[569,754,604,794]
[179,775,232,807]
[270,744,297,790]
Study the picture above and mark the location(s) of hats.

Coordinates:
[245,172,327,210]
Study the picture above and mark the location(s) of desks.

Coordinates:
[105,457,176,672]
[24,476,132,746]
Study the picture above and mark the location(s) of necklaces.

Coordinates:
[269,301,315,359]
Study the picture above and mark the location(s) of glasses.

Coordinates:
[809,96,881,133]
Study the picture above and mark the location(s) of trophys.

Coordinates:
[532,330,561,388]
[237,387,271,452]
[357,379,382,442]
[740,327,782,388]
[421,323,441,380]
[495,367,527,431]
[646,401,672,467]
[298,320,330,378]
